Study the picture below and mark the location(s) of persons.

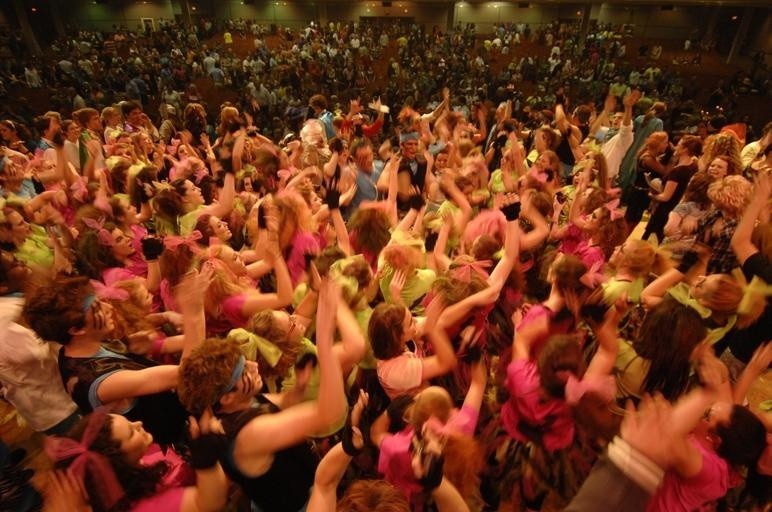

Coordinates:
[0,1,771,512]
